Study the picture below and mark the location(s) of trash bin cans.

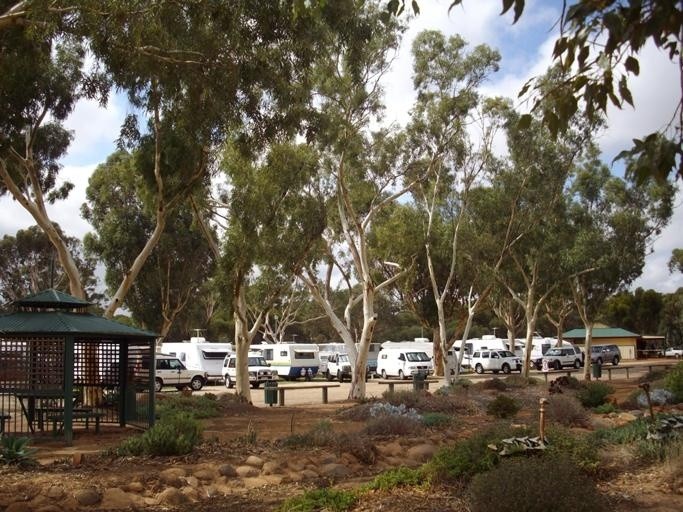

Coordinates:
[592,363,601,381]
[413,374,424,391]
[264,379,279,406]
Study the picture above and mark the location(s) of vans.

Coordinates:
[375,348,433,378]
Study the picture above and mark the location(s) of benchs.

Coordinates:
[643,364,673,373]
[0,406,106,437]
[601,366,635,381]
[536,369,579,382]
[377,379,439,393]
[257,381,341,403]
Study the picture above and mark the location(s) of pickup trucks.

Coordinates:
[656,346,683,359]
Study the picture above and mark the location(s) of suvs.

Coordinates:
[133,353,209,390]
[324,353,369,382]
[536,345,582,370]
[222,351,279,388]
[469,347,522,374]
[589,343,622,365]
